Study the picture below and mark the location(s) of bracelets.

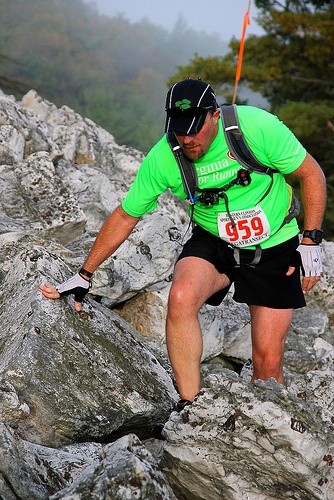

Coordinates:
[79,268,93,278]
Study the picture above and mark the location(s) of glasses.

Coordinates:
[165,103,216,119]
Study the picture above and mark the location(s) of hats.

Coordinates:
[163,78,217,135]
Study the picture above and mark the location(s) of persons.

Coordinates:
[39,79,327,442]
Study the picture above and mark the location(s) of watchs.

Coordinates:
[303,229,324,243]
